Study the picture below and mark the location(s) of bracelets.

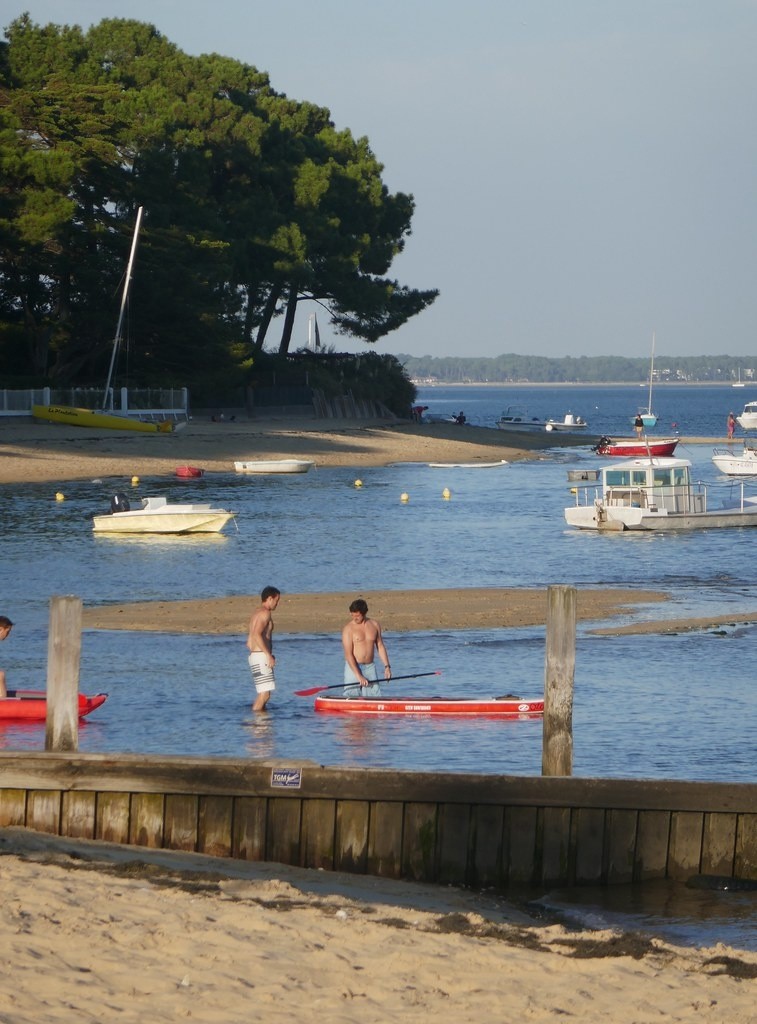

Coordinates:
[385,665,391,668]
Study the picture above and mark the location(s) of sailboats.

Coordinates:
[629,332,658,427]
[732,367,745,387]
[32,207,173,433]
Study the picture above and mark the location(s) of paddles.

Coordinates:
[293,671,442,697]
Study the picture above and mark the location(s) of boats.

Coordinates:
[495,405,588,432]
[176,466,205,477]
[314,694,544,715]
[92,493,240,533]
[423,413,460,423]
[234,459,314,473]
[565,435,757,530]
[0,689,108,719]
[736,401,757,429]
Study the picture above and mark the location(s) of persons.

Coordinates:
[412,406,428,422]
[0,616,13,698]
[457,411,466,423]
[342,598,391,696]
[727,412,736,439]
[634,414,644,437]
[246,586,280,710]
